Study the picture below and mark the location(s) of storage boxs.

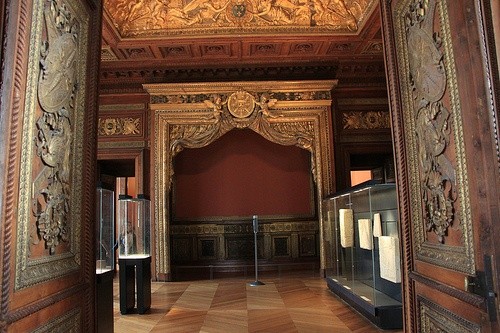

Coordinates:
[118,199,152,259]
[325,182,402,308]
[94,187,115,274]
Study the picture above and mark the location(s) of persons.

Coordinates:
[111,221,138,255]
